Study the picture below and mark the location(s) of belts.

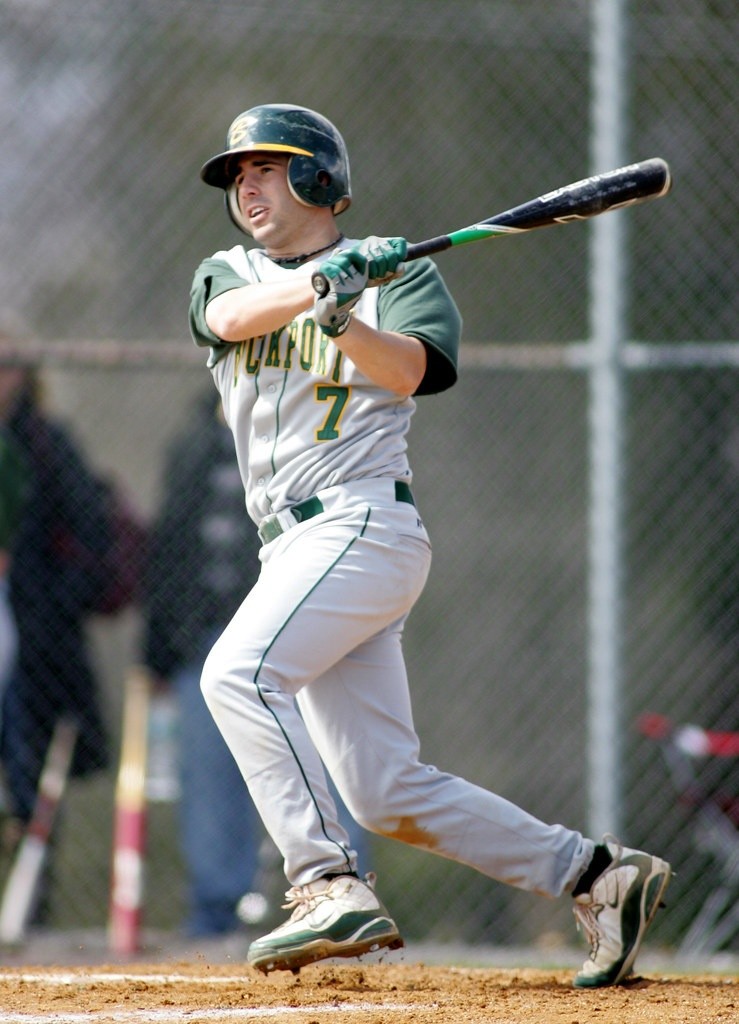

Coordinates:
[262,479,417,545]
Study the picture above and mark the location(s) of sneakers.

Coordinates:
[246,872,403,976]
[571,834,677,990]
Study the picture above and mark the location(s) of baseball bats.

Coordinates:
[107,658,151,957]
[1,717,79,947]
[308,155,674,298]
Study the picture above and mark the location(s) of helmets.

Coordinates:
[200,102,352,237]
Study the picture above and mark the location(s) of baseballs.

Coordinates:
[234,890,268,924]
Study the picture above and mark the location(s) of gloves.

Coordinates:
[354,235,409,288]
[312,247,370,337]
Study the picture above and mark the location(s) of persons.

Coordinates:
[137,382,363,960]
[0,310,110,934]
[191,102,673,987]
